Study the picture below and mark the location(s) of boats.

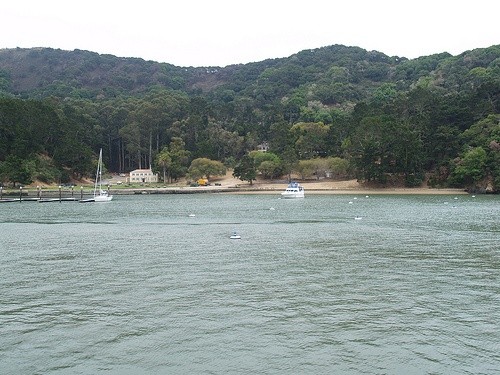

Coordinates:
[281,187,304,198]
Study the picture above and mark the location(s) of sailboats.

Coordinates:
[94,148,113,202]
